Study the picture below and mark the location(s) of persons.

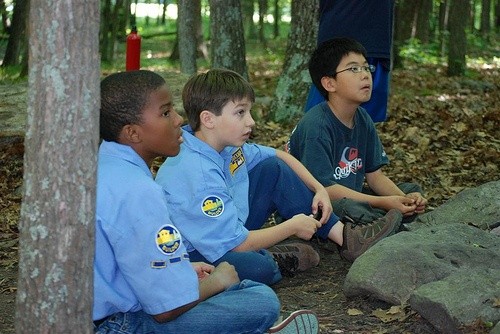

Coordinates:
[288,38,427,232]
[92,70,319,334]
[305,0,393,123]
[155,68,403,284]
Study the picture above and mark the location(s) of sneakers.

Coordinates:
[267,243,320,276]
[338,209,403,263]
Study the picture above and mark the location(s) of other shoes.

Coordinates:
[265,310,319,334]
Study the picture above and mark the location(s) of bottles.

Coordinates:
[126,27,141,71]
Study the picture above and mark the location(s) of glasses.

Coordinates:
[332,65,375,76]
